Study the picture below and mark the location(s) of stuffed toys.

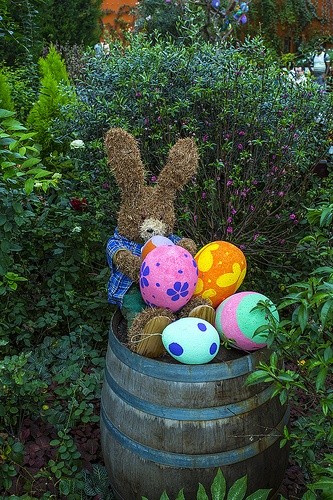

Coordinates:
[105,129,217,358]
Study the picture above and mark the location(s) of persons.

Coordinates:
[310,45,330,86]
[290,66,305,84]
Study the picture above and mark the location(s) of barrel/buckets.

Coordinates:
[101,307,291,500]
